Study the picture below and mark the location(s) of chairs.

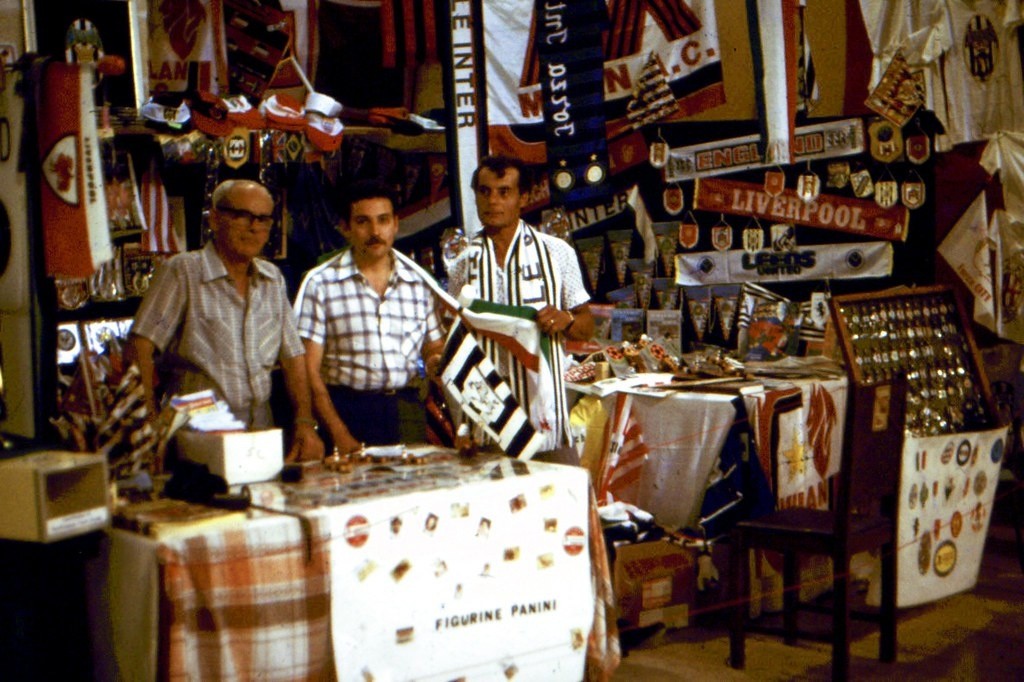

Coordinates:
[721,370,910,682]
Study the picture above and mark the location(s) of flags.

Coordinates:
[458,296,565,419]
[696,394,785,550]
[33,58,116,278]
[592,390,650,504]
[433,310,550,464]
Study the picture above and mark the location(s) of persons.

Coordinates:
[434,153,602,463]
[292,172,448,453]
[128,176,329,466]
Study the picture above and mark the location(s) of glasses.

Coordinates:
[214,206,276,230]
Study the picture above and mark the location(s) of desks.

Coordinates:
[564,356,851,531]
[85,439,625,682]
